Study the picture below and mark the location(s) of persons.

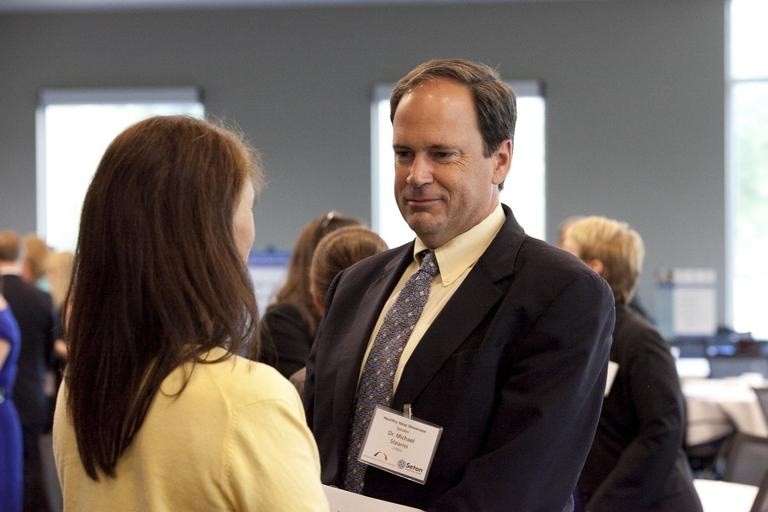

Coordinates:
[306,57,616,512]
[52,115,331,512]
[246,214,363,380]
[288,227,389,410]
[0,230,75,512]
[562,217,703,512]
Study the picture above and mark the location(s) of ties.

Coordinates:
[344,248,438,495]
